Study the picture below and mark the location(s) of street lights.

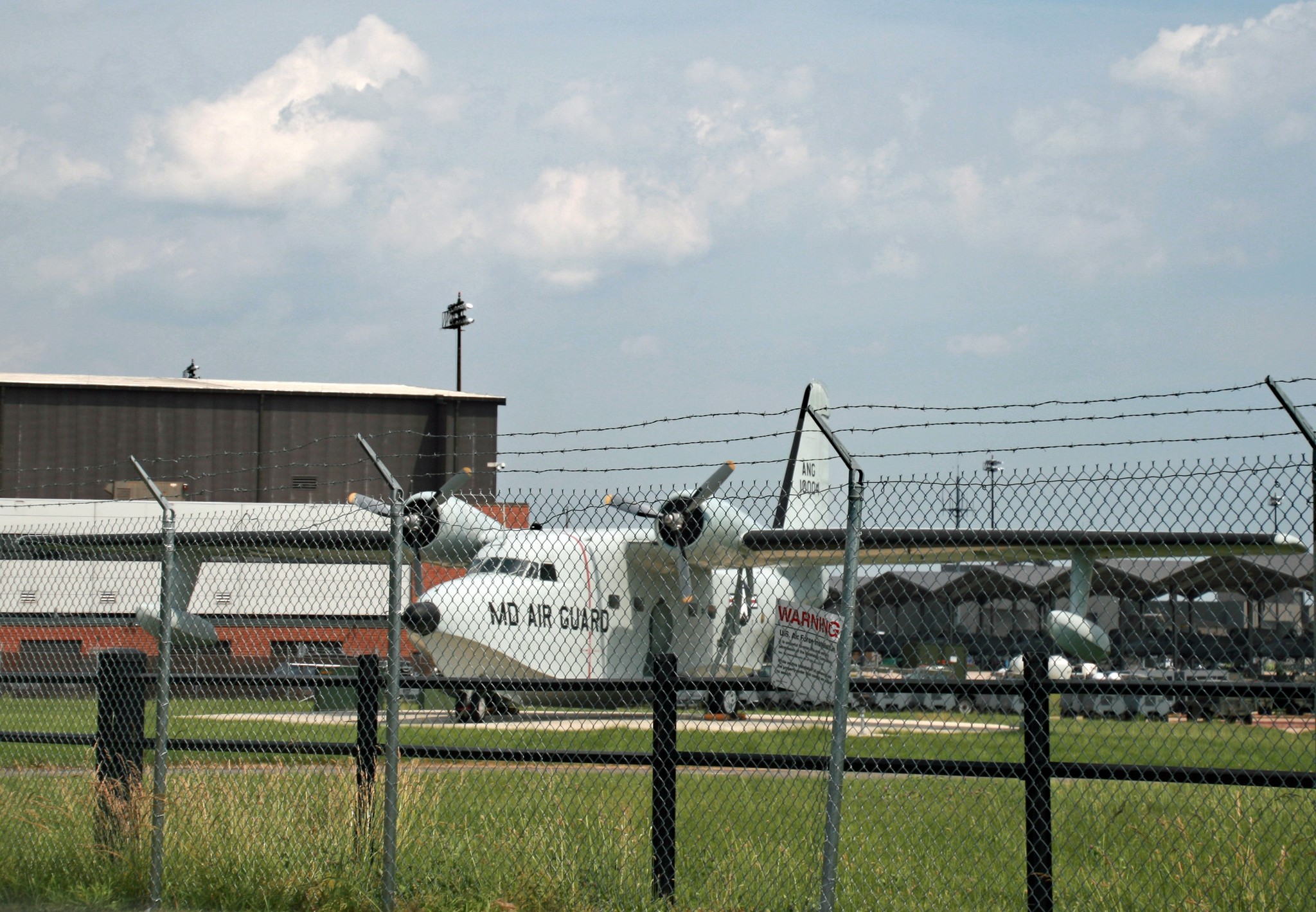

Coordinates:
[1268,493,1284,532]
[983,455,1005,529]
[440,292,475,392]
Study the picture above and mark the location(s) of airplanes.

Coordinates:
[347,377,1309,725]
